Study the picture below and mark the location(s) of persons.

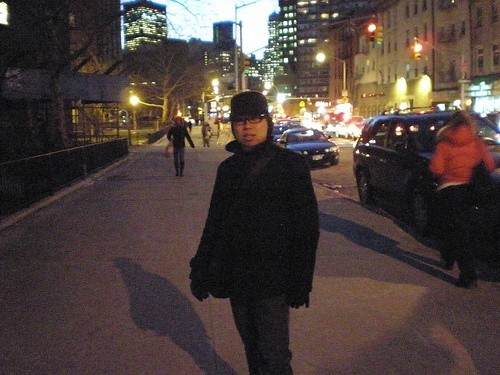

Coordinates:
[186,90,322,375]
[426,108,500,288]
[188,120,193,133]
[201,122,212,148]
[167,115,196,177]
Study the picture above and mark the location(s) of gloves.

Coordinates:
[287,275,312,309]
[189,258,210,302]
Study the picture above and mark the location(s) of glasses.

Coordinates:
[233,116,264,125]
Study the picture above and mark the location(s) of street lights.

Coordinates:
[315,52,347,102]
[130,96,138,130]
[415,38,466,113]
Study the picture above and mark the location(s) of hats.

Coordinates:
[229,91,270,119]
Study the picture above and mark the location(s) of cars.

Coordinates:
[272,120,339,168]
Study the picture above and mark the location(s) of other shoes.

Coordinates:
[456,273,477,289]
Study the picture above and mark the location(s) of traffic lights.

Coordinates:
[366,24,384,47]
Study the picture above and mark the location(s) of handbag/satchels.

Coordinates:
[164,140,173,158]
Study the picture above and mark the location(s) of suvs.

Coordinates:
[352,111,500,232]
